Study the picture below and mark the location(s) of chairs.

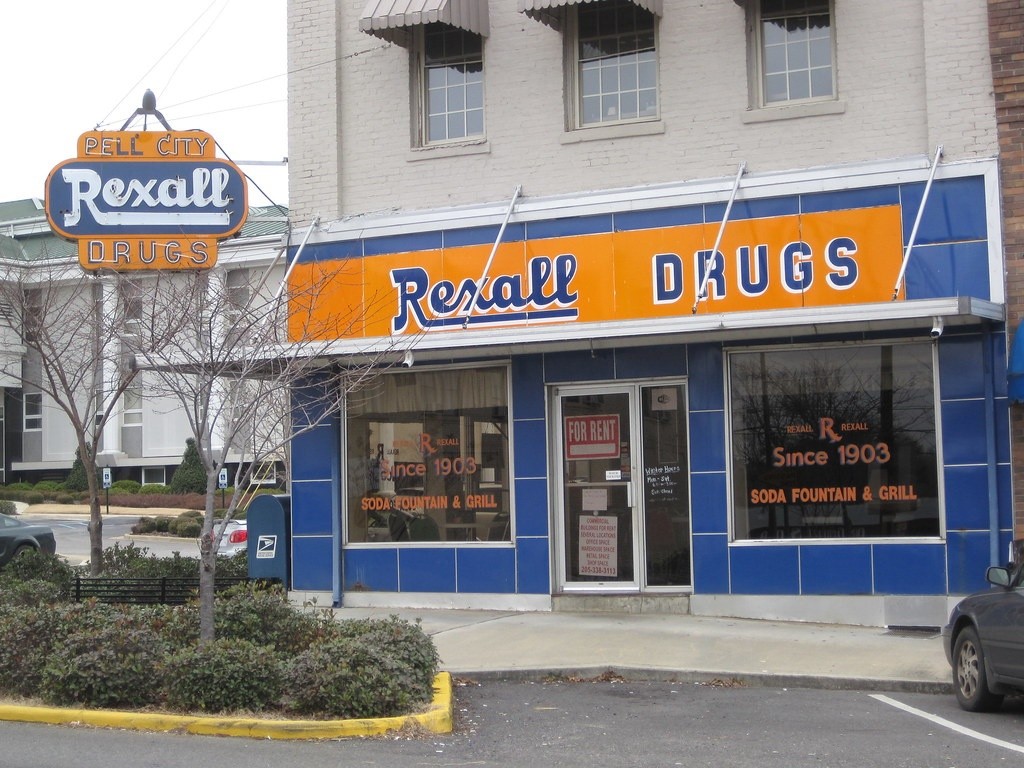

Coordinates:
[388,510,439,541]
[487,511,510,541]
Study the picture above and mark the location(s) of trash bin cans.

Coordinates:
[247,494,291,591]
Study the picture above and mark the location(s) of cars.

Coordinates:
[196,519,248,558]
[0,512,57,573]
[941,559,1024,713]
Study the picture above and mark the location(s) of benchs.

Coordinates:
[71,577,279,608]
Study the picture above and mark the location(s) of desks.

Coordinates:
[445,522,505,541]
